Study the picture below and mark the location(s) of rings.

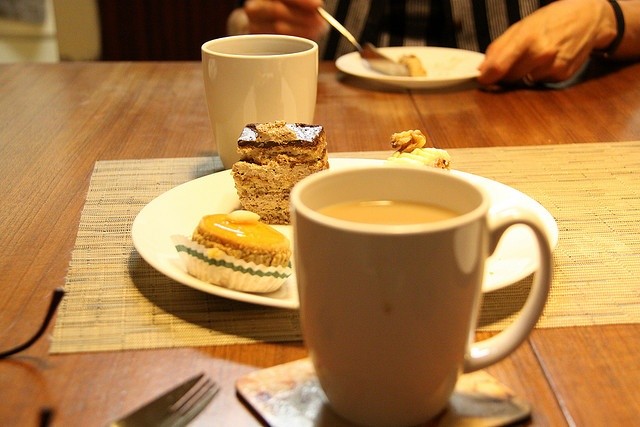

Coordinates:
[523,74,533,86]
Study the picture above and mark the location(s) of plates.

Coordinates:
[334,46,488,91]
[130,157,558,312]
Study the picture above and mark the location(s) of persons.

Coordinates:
[226,0,640,92]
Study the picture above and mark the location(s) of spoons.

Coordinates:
[315,5,408,75]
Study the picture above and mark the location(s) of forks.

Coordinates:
[107,372,220,426]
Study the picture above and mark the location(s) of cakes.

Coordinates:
[382,129,450,172]
[230,120,330,225]
[397,55,428,78]
[169,210,294,293]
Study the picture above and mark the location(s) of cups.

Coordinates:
[201,34,318,184]
[289,162,553,427]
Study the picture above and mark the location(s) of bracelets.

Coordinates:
[594,0,624,56]
